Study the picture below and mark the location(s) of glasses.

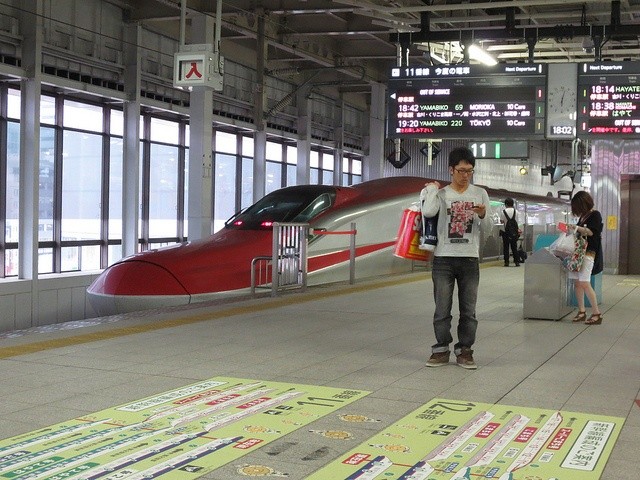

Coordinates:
[453,166,474,175]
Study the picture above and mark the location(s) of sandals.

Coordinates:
[585,313,603,325]
[571,311,587,323]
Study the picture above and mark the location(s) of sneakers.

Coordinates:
[425,349,452,367]
[504,263,509,267]
[457,349,477,370]
[516,262,520,266]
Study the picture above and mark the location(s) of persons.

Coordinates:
[500,198,520,267]
[567,191,603,323]
[422,148,493,368]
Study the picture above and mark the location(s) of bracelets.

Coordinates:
[477,213,485,219]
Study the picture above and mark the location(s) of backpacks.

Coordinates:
[503,209,519,239]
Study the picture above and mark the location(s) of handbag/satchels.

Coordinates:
[563,227,589,272]
[392,185,431,262]
[419,186,437,252]
[549,226,580,259]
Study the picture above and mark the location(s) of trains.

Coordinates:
[85,176,574,316]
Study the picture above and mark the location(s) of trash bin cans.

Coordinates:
[523,248,573,321]
